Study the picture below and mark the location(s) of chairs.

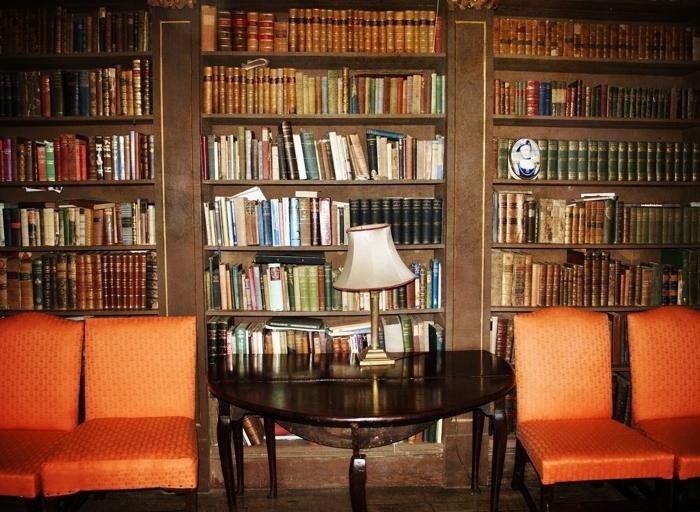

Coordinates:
[40,316,201,512]
[626,305,700,512]
[512,305,675,512]
[0,312,84,512]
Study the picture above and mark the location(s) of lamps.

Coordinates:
[332,223,416,367]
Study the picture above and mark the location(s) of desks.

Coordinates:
[204,350,516,512]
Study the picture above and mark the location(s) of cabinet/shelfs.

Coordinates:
[0,0,165,316]
[488,1,699,442]
[193,0,454,491]
